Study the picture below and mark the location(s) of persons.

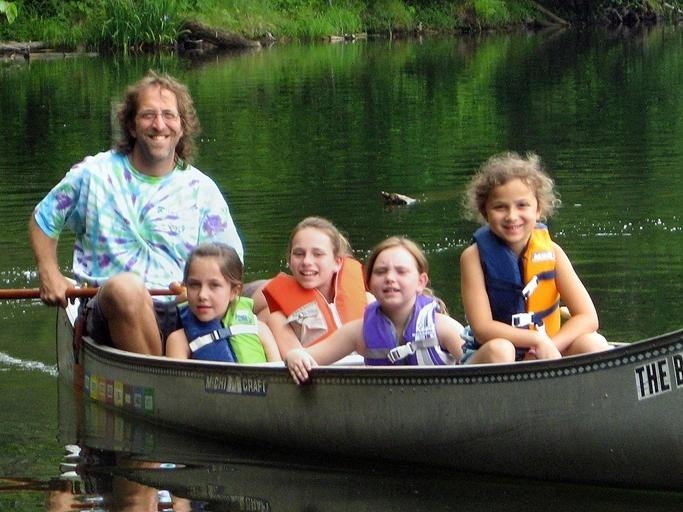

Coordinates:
[32,69,244,358]
[262,217,375,386]
[307,234,464,365]
[460,151,609,362]
[164,243,282,364]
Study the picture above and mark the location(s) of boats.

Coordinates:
[55,272,683,492]
